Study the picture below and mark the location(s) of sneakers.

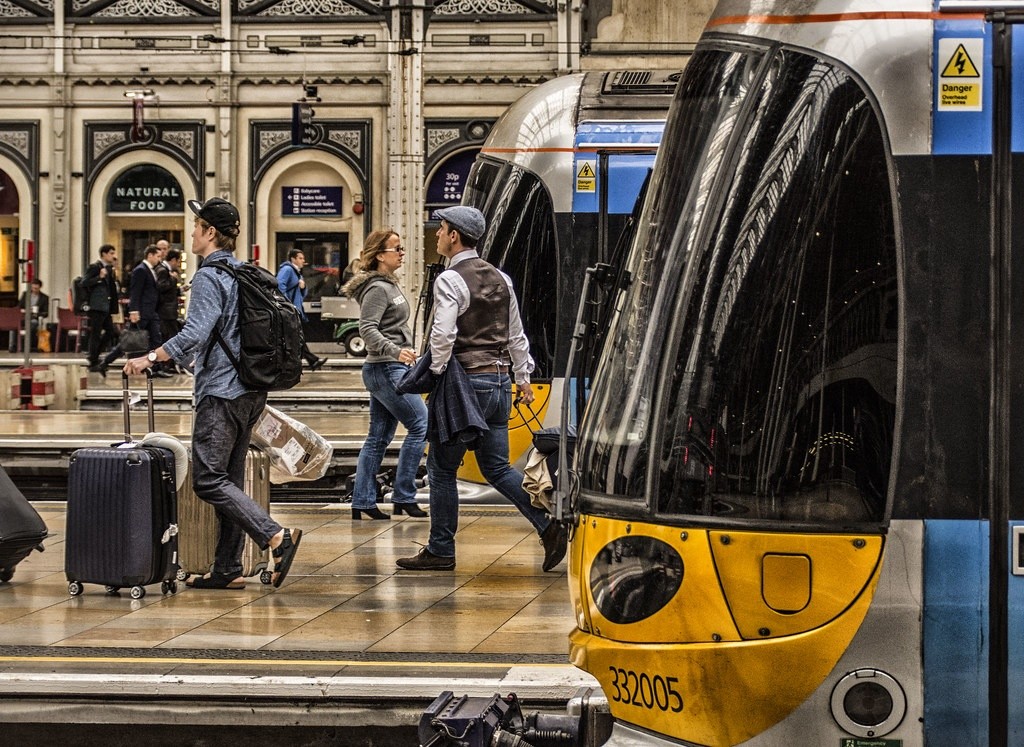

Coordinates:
[396,540,456,570]
[539,518,568,572]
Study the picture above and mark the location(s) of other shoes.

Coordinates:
[312,357,328,370]
[165,364,186,374]
[90,363,109,371]
[152,370,173,378]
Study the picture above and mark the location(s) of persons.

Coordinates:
[122,198,302,589]
[334,258,361,297]
[79,239,191,379]
[396,205,569,571]
[337,230,428,520]
[276,249,328,372]
[9,279,48,353]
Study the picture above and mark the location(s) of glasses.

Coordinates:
[377,246,405,252]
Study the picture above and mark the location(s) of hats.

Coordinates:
[188,197,241,238]
[434,205,486,240]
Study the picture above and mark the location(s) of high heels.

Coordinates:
[351,506,391,521]
[393,501,428,518]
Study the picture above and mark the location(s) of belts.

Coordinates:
[464,365,511,374]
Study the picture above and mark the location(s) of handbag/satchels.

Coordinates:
[246,408,334,484]
[36,321,51,352]
[120,321,150,351]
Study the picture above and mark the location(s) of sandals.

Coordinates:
[270,528,302,586]
[186,566,246,589]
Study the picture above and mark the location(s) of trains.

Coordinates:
[376,70,685,508]
[413,1,1024,747]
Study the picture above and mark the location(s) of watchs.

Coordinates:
[148,350,158,365]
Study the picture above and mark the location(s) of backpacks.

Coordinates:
[197,260,303,391]
[72,262,103,316]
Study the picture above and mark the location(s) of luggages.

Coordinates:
[45,297,67,353]
[63,366,273,597]
[0,464,49,582]
